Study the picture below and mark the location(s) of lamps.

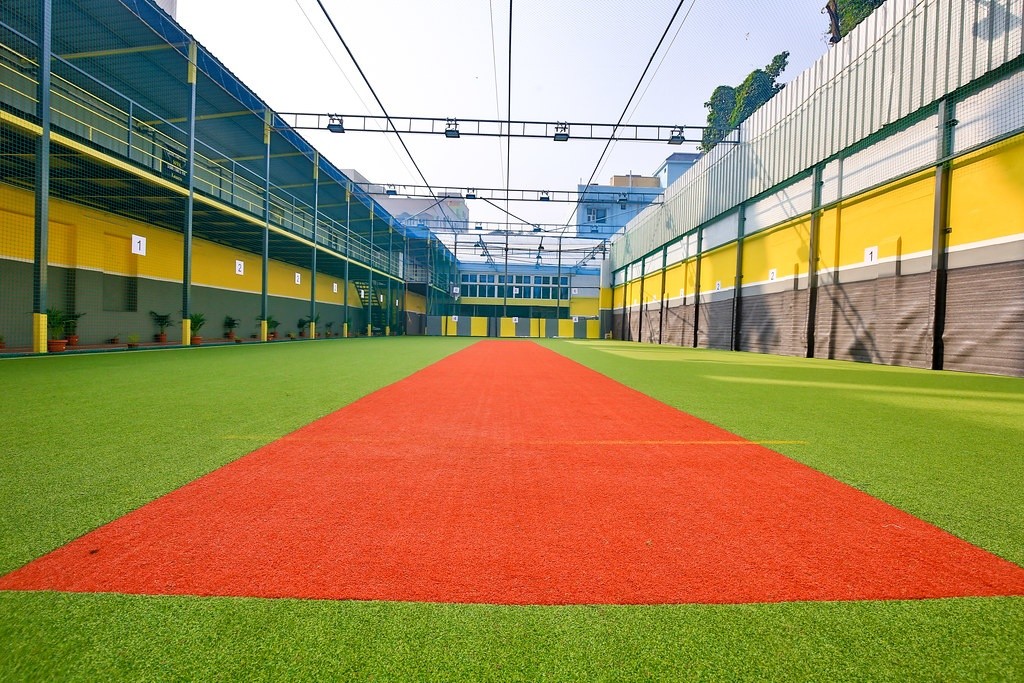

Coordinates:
[445,124,459,138]
[540,196,549,201]
[387,190,396,195]
[466,195,475,199]
[328,118,345,133]
[617,198,628,202]
[418,224,599,270]
[554,127,569,141]
[667,130,685,144]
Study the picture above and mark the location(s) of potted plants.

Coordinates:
[126,334,140,348]
[151,311,174,343]
[26,306,86,353]
[179,310,208,345]
[223,316,398,344]
[0,336,8,349]
[109,333,121,344]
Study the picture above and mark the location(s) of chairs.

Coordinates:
[605,330,612,339]
